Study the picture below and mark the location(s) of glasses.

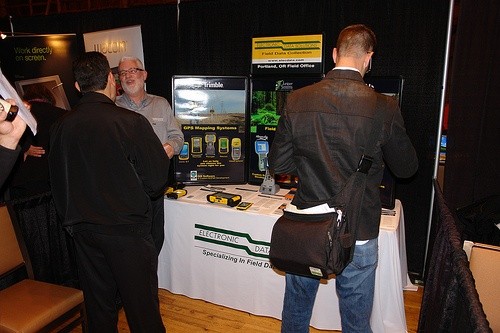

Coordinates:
[119,67,144,76]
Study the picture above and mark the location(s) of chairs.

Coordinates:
[0,203,89,333]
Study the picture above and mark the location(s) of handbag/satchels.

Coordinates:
[268,172,365,280]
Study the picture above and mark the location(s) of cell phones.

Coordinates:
[236,202,253,210]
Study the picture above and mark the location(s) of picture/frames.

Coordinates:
[16,74,72,113]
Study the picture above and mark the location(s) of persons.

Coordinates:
[0,98,31,189]
[27,50,170,333]
[269,24,418,333]
[2,83,72,290]
[114,54,183,159]
[0,145,47,200]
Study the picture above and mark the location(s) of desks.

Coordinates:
[152,184,418,333]
